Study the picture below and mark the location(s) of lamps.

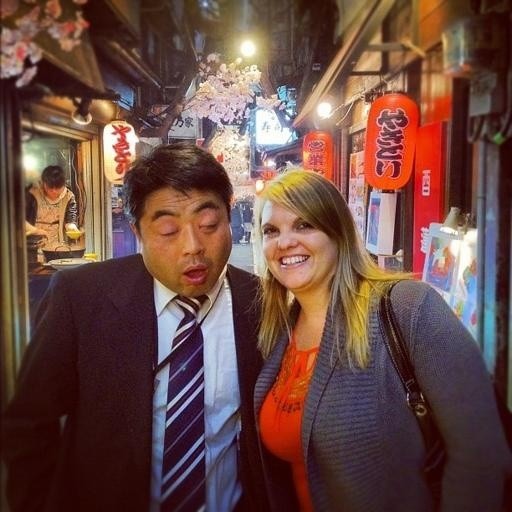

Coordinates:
[67,95,95,125]
[439,206,479,238]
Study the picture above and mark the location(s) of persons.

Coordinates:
[0,140,304,510]
[26,165,78,256]
[253,165,511,510]
[230,200,252,245]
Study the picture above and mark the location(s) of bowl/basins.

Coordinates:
[84,253,98,261]
[43,258,89,273]
[65,230,84,242]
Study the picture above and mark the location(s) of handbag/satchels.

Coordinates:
[422,379,511,496]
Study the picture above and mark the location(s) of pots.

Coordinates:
[41,243,86,262]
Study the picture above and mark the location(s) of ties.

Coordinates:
[161,296,209,512]
